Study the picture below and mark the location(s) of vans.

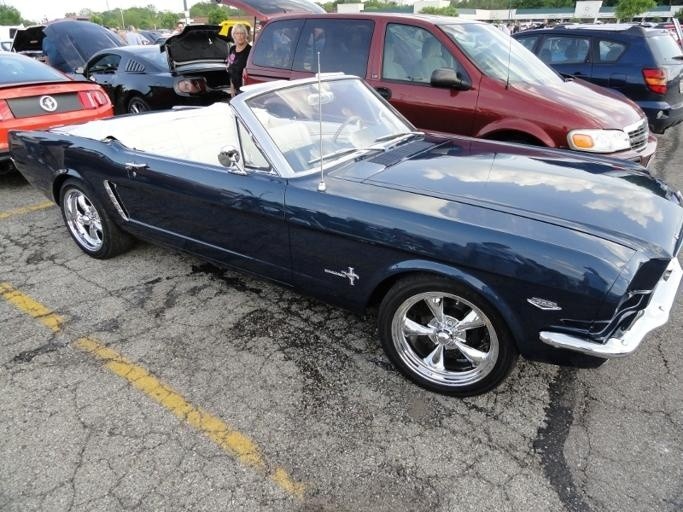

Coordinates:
[215,1,659,167]
[510,23,682,135]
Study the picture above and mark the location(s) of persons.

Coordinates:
[502,19,550,38]
[172,23,184,36]
[224,22,252,99]
[125,25,141,46]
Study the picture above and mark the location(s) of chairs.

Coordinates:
[382,39,449,81]
[540,46,622,63]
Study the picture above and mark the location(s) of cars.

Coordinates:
[0,19,249,160]
[9,75,683,396]
